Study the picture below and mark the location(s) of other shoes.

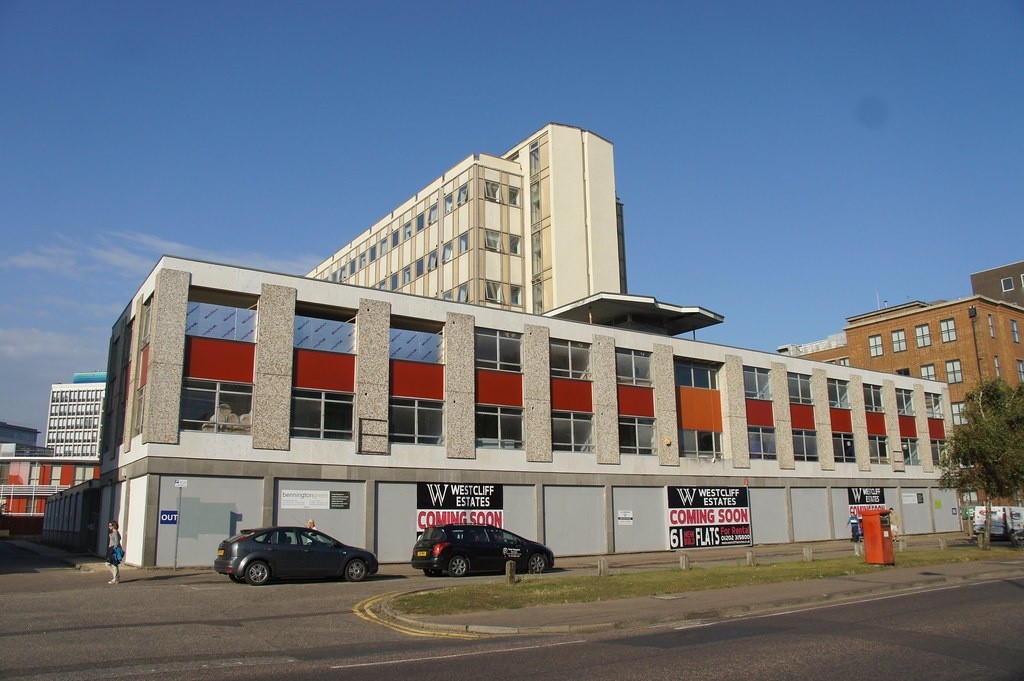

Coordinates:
[108,580,119,584]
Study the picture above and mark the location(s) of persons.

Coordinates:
[846,512,861,543]
[306,518,318,545]
[889,508,900,542]
[107,520,121,585]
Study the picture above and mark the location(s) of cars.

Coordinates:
[966,508,975,520]
[411,523,555,578]
[214,526,380,586]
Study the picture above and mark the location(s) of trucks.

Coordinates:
[972,505,1024,541]
